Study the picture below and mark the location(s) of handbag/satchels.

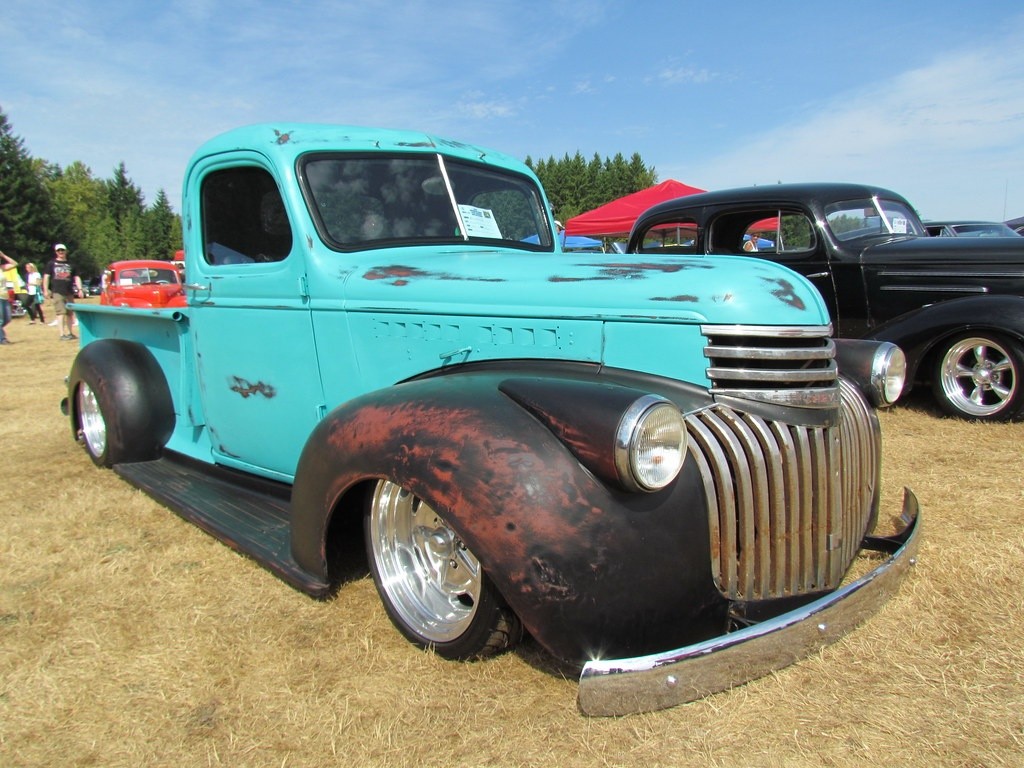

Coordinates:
[35,284,45,304]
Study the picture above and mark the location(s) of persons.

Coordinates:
[742,231,760,252]
[42,244,84,340]
[20,262,48,325]
[0,250,20,345]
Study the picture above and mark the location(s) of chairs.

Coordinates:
[222,188,458,262]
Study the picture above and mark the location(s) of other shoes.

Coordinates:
[0,338,11,345]
[28,321,47,325]
[60,333,79,341]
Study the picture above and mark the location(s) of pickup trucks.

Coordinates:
[63,123,920,718]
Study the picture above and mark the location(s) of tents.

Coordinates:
[560,180,788,260]
[519,229,777,253]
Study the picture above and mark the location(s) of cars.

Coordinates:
[100,260,189,308]
[623,183,1024,423]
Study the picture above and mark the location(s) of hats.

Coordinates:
[55,243,66,252]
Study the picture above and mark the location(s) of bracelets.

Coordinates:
[78,288,83,291]
